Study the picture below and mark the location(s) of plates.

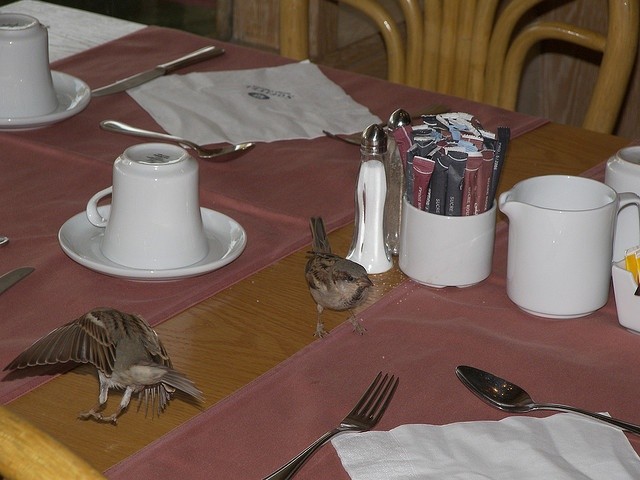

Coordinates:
[0,69,92,132]
[57,204,248,284]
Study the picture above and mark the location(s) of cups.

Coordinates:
[86,142,209,272]
[603,145,640,262]
[498,173,640,319]
[397,190,498,290]
[0,12,60,119]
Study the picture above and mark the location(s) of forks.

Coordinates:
[322,102,451,146]
[258,370,400,480]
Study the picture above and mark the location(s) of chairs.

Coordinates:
[279,0,640,137]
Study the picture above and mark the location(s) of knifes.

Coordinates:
[0,265,36,295]
[91,44,226,97]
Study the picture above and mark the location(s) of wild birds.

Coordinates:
[303,216,375,340]
[2,306,207,426]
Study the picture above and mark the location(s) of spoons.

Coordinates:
[455,364,640,438]
[98,118,256,164]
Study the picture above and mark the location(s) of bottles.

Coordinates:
[383,107,413,257]
[345,123,395,277]
